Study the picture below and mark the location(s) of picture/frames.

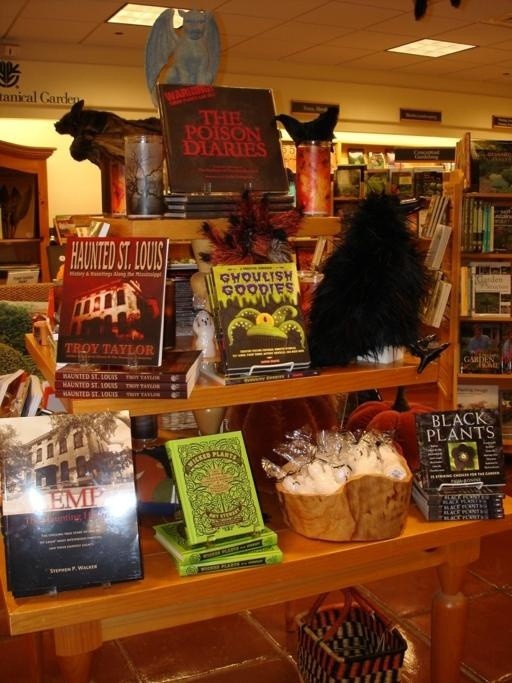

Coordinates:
[0,140,57,284]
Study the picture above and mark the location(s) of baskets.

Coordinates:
[295,588,408,683]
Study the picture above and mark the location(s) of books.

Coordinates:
[1,83,512,596]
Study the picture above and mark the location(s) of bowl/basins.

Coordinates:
[276,445,412,542]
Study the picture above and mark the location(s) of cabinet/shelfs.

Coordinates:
[438,179,512,457]
[330,144,456,216]
[0,217,512,683]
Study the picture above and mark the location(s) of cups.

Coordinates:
[296,141,331,217]
[131,414,157,445]
[109,160,126,217]
[124,135,166,220]
